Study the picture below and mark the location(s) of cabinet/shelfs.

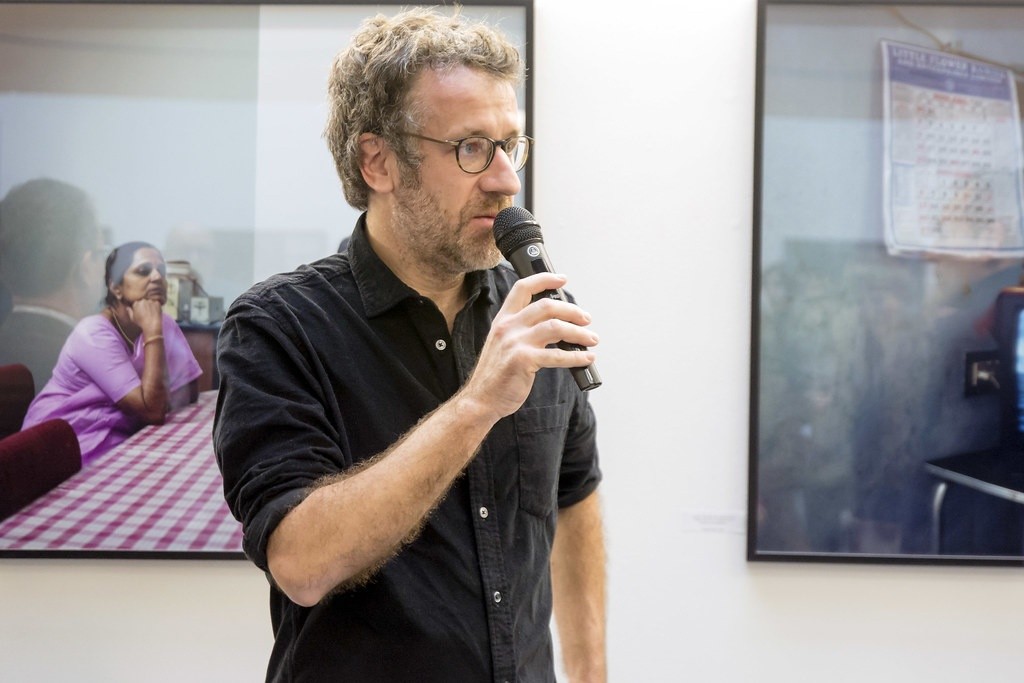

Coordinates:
[177,319,224,391]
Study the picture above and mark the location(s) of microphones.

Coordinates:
[491,206,603,393]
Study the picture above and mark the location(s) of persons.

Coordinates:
[212,11,609,683]
[21,244,202,470]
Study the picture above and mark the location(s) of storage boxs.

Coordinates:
[189,294,225,325]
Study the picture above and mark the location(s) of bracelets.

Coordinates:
[144,335,164,349]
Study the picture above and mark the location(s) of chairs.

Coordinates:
[0,363,35,440]
[0,417,82,524]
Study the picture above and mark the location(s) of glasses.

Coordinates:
[363,123,535,174]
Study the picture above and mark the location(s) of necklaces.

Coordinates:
[111,310,135,346]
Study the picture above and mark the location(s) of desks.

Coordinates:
[0,389,243,552]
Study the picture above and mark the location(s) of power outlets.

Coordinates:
[965,348,1003,397]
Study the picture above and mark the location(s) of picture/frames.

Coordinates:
[0,0,539,558]
[748,0,1024,568]
